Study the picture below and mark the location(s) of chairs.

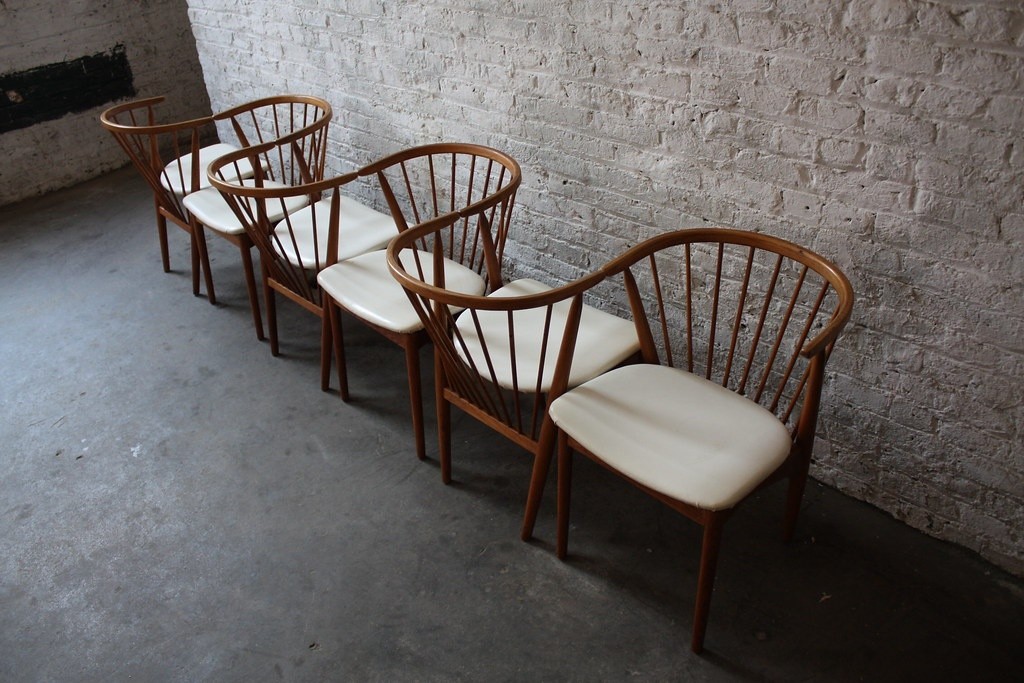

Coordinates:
[101,95,854,653]
